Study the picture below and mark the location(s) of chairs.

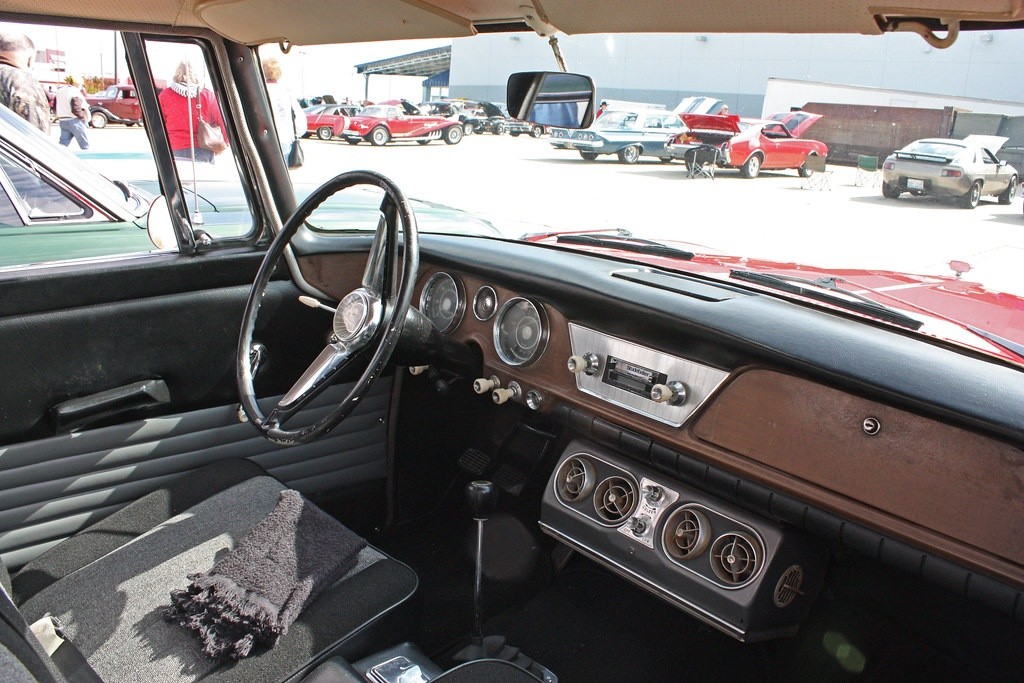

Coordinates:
[855,155,882,188]
[0,457,419,683]
[685,144,720,179]
[800,155,833,191]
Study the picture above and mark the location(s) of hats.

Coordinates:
[721,105,730,110]
[600,102,609,107]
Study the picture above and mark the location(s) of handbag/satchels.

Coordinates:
[289,106,304,169]
[196,86,229,152]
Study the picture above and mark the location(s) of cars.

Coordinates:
[881,133,1018,209]
[460,99,551,137]
[0,100,503,270]
[342,103,461,146]
[664,106,828,176]
[550,109,690,161]
[301,101,459,141]
[85,84,142,129]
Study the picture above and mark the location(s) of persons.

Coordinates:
[718,104,727,115]
[159,58,229,166]
[263,58,306,167]
[299,97,363,108]
[0,29,92,149]
[596,102,608,119]
